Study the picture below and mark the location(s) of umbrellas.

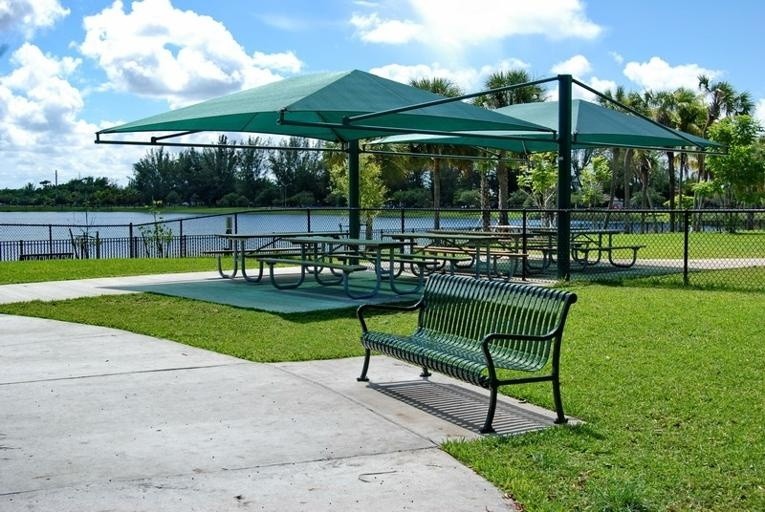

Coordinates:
[92,68,558,265]
[359,98,728,280]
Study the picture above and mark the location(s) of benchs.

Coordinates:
[356,273,579,434]
